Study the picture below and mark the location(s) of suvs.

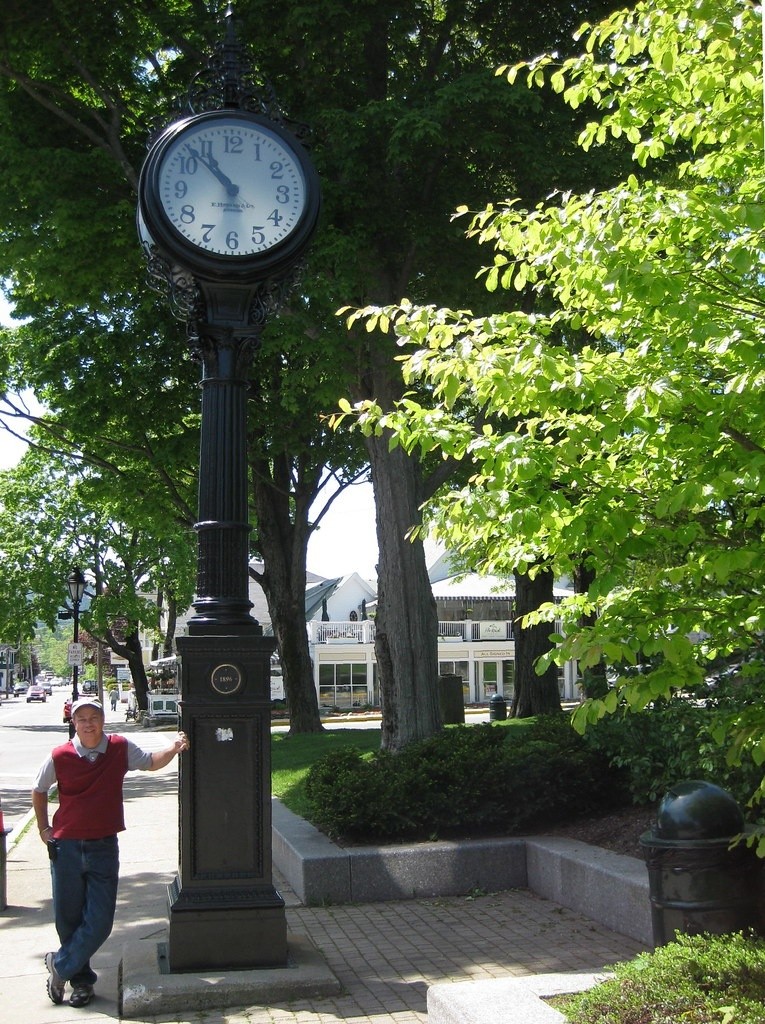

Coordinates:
[82,679,98,696]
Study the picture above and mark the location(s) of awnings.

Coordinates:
[150,656,176,667]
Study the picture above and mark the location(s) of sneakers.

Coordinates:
[44,952,66,1004]
[69,984,94,1007]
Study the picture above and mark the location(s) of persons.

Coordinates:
[109,687,118,711]
[33,697,190,1008]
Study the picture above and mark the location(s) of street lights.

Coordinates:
[57,563,89,741]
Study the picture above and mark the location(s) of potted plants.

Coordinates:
[466,609,474,614]
[437,632,463,642]
[368,612,376,618]
[326,636,358,644]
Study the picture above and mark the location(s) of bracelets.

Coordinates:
[39,826,51,834]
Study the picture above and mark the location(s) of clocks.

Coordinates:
[143,110,320,275]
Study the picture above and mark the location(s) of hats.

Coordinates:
[70,696,103,717]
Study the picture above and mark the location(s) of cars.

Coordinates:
[26,686,47,703]
[12,681,30,697]
[50,678,70,687]
[40,682,53,696]
[62,696,83,722]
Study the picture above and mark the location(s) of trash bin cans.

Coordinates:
[639,780,759,955]
